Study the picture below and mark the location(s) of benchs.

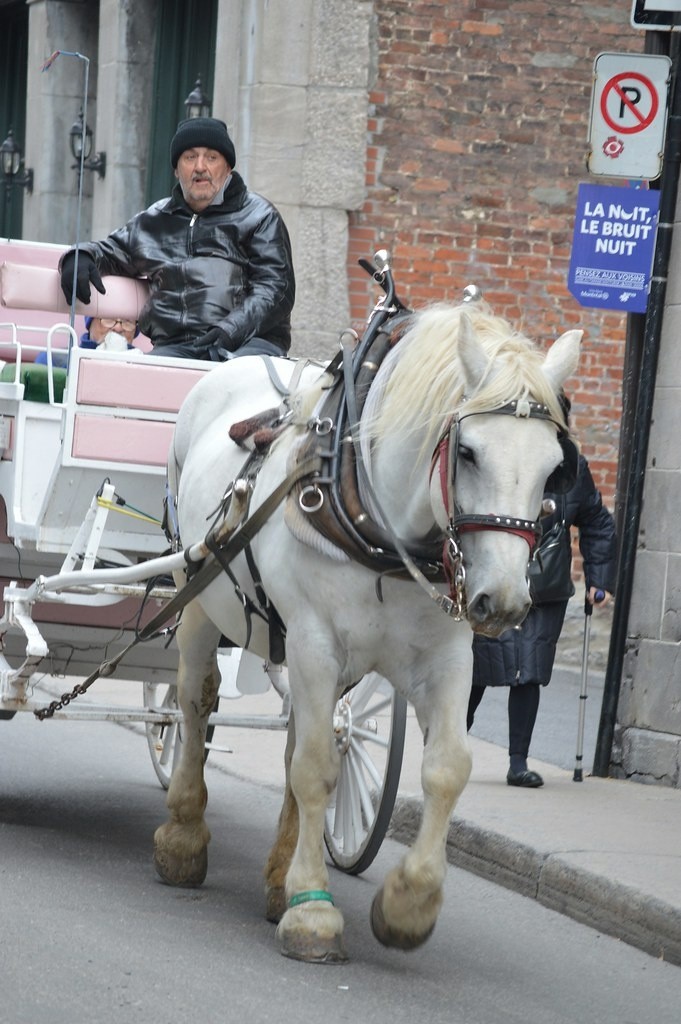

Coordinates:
[0,237,224,477]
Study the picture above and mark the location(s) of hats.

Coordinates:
[171,117,235,169]
[85,316,141,338]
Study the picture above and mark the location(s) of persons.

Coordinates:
[34,316,140,369]
[466,384,619,787]
[58,117,295,363]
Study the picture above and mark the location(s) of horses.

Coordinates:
[151,296,587,966]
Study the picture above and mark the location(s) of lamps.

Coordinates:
[184,71,212,118]
[0,125,33,194]
[69,105,106,180]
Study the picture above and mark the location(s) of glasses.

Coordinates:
[100,318,137,332]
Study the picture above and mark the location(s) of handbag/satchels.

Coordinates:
[528,520,576,602]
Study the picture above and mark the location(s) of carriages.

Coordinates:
[0,50,585,968]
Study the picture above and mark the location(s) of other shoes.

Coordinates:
[507,767,544,787]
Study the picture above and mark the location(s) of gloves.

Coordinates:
[61,250,106,305]
[194,326,234,361]
